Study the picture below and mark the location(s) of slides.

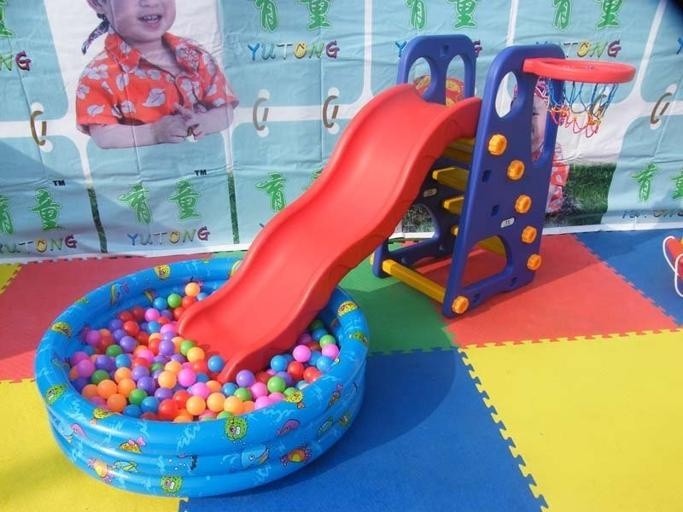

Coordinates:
[176,83,482,390]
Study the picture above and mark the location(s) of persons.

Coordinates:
[508,81,569,215]
[72,0,239,149]
